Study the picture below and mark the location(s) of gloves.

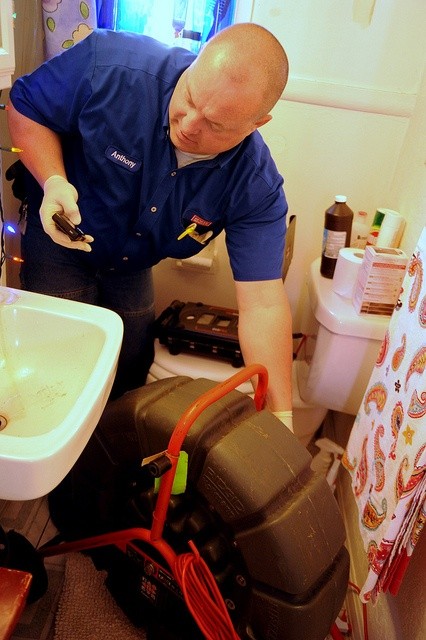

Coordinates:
[273,410,294,433]
[41,175,94,252]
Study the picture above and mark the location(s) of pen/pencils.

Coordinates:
[174,223,198,241]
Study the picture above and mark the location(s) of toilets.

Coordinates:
[146,257,393,449]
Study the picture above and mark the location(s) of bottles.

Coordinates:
[350,210,370,249]
[319,194,355,280]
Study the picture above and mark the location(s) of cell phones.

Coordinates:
[50,210,84,241]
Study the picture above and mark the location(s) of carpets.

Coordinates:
[54,557,139,636]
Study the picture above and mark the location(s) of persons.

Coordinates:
[8,22,294,558]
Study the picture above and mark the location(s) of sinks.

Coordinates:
[0,286,123,503]
